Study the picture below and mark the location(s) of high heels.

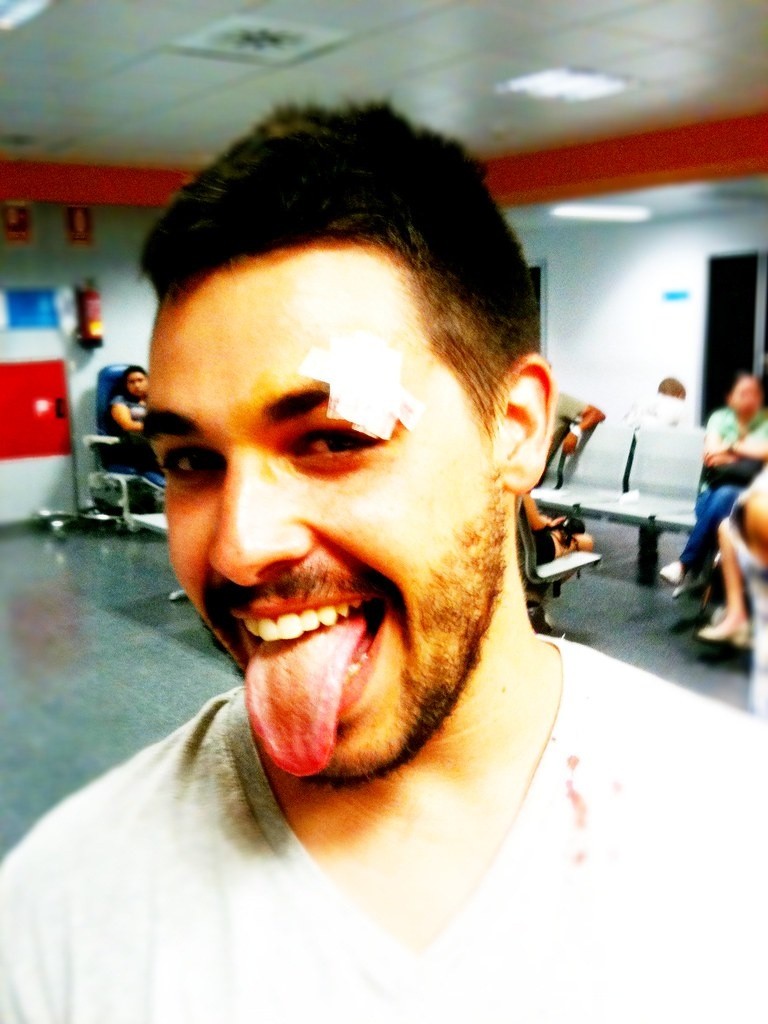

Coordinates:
[699,606,749,647]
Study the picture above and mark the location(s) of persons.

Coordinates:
[516,492,595,641]
[659,373,768,714]
[0,98,768,1024]
[97,365,166,515]
[618,377,693,432]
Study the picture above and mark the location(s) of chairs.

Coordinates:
[583,425,708,526]
[544,422,640,517]
[530,437,566,506]
[515,493,602,598]
[654,512,698,534]
[82,361,166,532]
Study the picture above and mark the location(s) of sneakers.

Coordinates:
[660,562,685,586]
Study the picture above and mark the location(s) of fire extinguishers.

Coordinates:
[72,278,104,350]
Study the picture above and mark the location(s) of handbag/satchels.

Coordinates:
[550,516,585,547]
